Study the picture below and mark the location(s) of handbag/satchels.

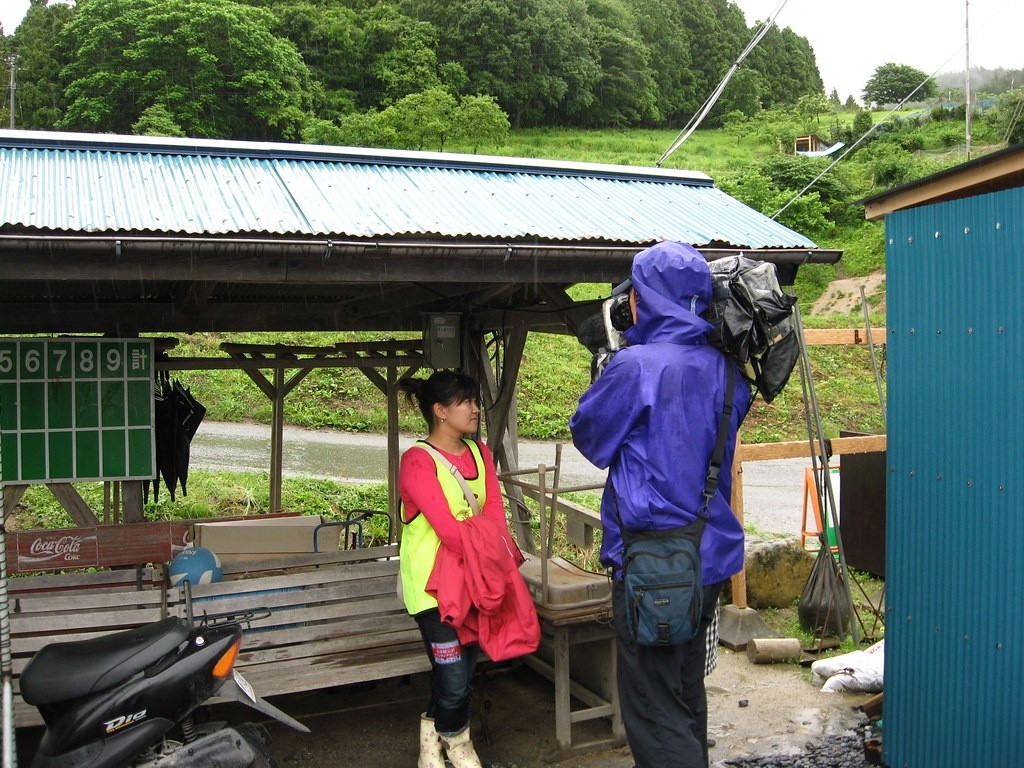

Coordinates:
[620,514,708,650]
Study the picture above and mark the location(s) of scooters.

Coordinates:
[0,608,313,768]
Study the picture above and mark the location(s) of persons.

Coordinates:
[569,238,754,768]
[398,369,511,768]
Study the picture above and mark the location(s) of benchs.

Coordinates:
[0,511,496,747]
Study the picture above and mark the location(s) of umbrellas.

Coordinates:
[143,352,206,504]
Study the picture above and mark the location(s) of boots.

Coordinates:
[417,712,446,768]
[438,721,483,768]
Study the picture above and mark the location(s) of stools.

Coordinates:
[497,444,613,611]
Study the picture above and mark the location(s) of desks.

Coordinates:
[517,603,628,765]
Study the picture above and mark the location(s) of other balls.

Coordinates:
[168,547,222,586]
